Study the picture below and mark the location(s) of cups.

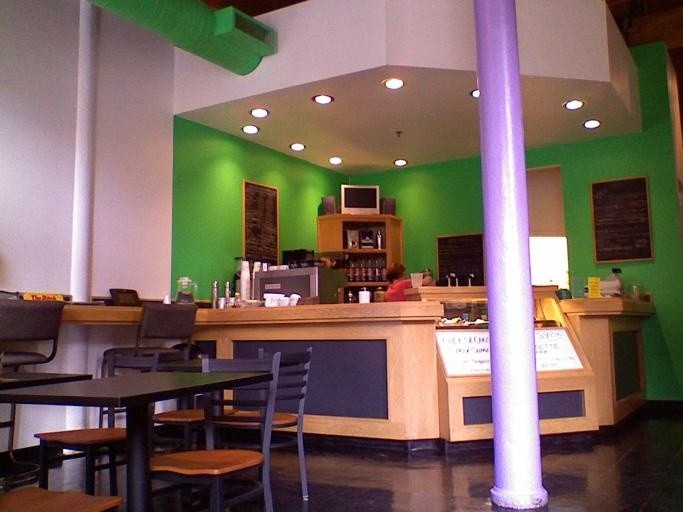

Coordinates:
[163,294,171,305]
[240,261,261,300]
[279,298,298,306]
[229,298,235,305]
[628,284,640,301]
[410,273,423,288]
[218,298,225,308]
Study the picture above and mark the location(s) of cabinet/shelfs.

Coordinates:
[317,214,403,304]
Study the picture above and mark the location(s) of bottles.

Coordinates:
[225,282,231,304]
[212,281,218,308]
[345,260,386,303]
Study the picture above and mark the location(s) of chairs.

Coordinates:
[0,488,123,512]
[152,353,237,451]
[0,300,66,490]
[33,354,160,497]
[216,346,312,502]
[98,303,198,428]
[148,352,273,512]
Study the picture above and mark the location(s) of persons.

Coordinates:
[386,263,433,302]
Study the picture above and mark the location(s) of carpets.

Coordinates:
[120,477,391,512]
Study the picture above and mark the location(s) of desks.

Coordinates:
[0,372,273,512]
[62,304,143,326]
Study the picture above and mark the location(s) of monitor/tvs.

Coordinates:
[341,184,380,214]
[254,267,318,305]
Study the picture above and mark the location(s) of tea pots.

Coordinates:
[175,277,197,304]
